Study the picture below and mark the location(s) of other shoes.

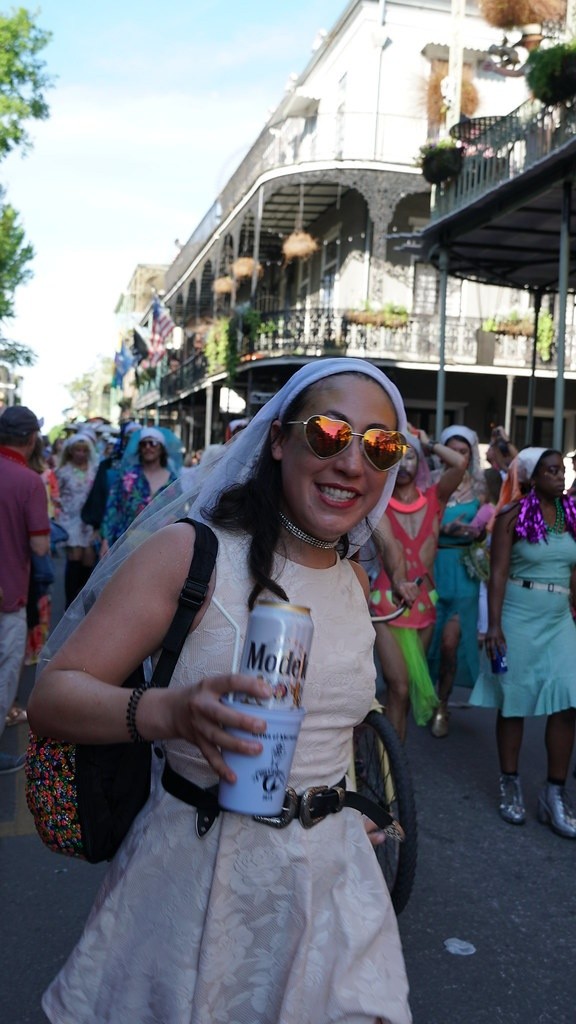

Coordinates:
[5,710,27,726]
[430,703,451,737]
[25,655,41,665]
[0,752,26,774]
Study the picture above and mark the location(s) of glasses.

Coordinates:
[285,415,412,472]
[140,440,159,447]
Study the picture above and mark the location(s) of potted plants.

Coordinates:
[412,139,466,183]
[344,302,408,329]
[480,311,536,338]
[525,37,576,107]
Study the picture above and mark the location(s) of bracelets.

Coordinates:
[426,440,440,455]
[126,683,165,744]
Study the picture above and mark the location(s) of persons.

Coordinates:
[165,338,206,396]
[483,23,559,159]
[351,425,576,844]
[26,357,412,1024]
[0,396,226,774]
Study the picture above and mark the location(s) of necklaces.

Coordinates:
[388,486,426,514]
[279,511,340,549]
[544,498,564,535]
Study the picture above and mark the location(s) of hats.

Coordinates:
[0,406,41,436]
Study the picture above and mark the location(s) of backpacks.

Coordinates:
[25,518,227,864]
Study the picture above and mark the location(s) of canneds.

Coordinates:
[232,596,313,711]
[491,641,508,673]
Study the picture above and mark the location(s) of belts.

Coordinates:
[510,578,572,596]
[160,761,395,831]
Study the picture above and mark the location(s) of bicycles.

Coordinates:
[353,711,421,916]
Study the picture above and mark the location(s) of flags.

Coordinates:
[111,295,176,389]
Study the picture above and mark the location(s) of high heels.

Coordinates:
[496,774,528,823]
[537,781,576,837]
